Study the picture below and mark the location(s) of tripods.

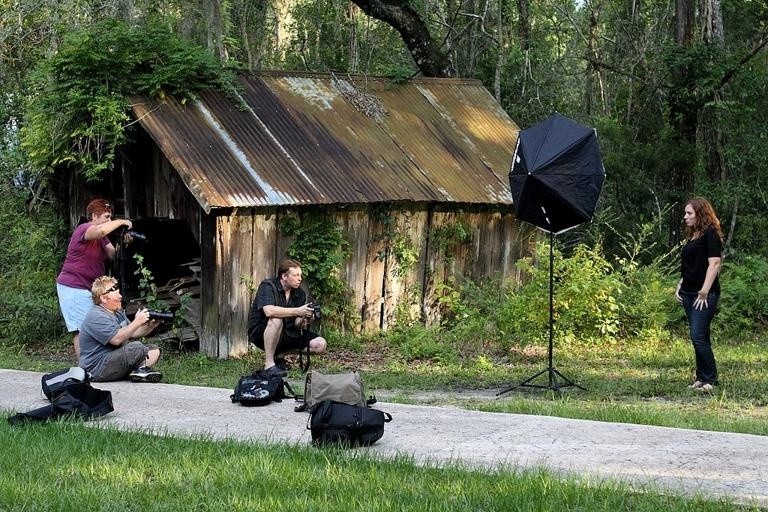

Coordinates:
[496,226,590,403]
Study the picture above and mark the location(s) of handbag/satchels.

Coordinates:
[5,377,115,427]
[230,375,296,406]
[294,370,377,412]
[40,366,93,401]
[306,399,393,450]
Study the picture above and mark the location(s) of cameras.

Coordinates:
[123,225,149,241]
[141,307,175,325]
[307,303,322,320]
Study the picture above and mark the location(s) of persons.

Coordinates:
[78,275,165,383]
[56,198,134,362]
[247,259,328,377]
[675,197,722,392]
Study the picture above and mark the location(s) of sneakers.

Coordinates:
[687,381,718,394]
[263,363,287,377]
[130,366,163,382]
